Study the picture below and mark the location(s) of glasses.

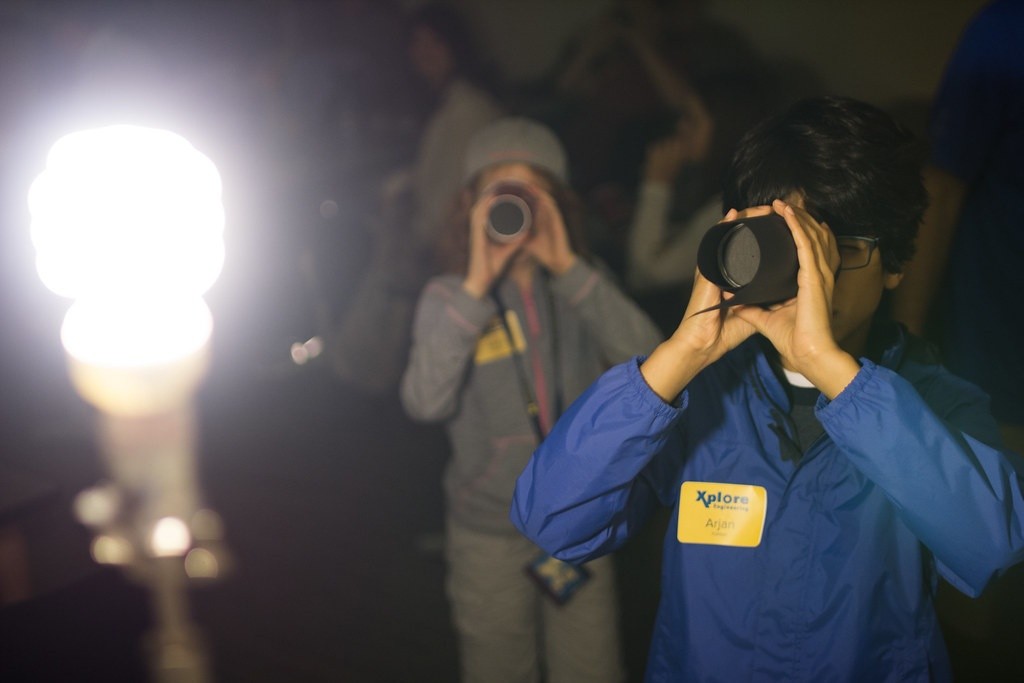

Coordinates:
[838,234,881,268]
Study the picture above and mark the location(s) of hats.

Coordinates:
[452,115,576,212]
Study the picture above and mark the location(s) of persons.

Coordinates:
[381,6,499,271]
[883,0,1024,644]
[400,118,664,683]
[508,96,1024,683]
[540,0,772,291]
